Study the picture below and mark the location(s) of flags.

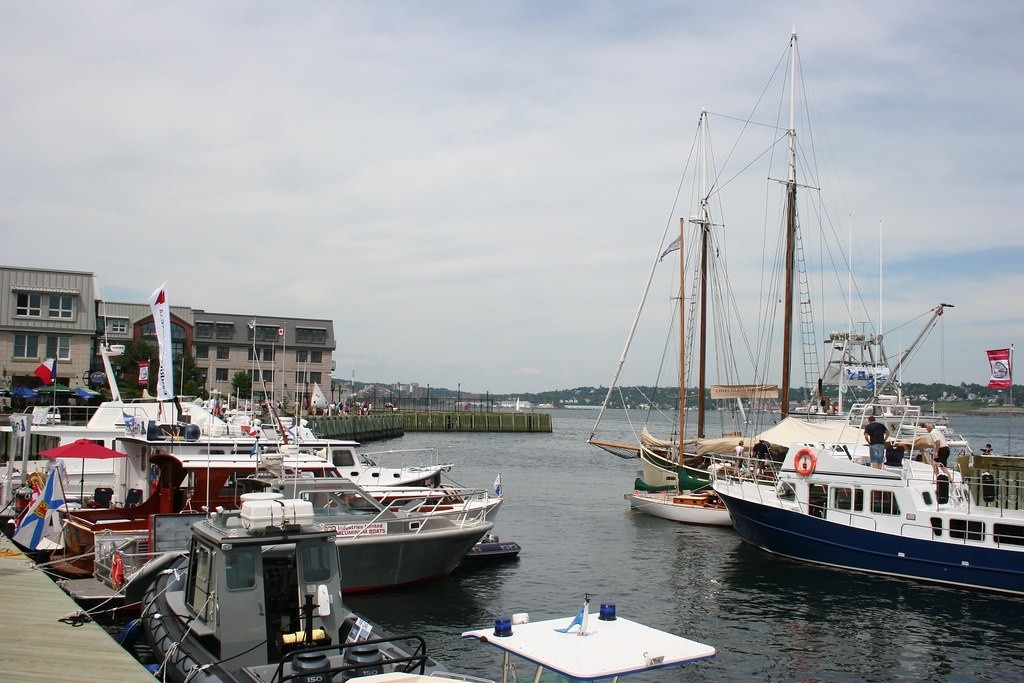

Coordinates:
[12,467,65,551]
[248,319,255,329]
[123,413,135,428]
[986,348,1011,389]
[278,328,284,335]
[493,474,501,496]
[157,402,161,421]
[138,361,148,385]
[147,281,173,400]
[553,607,584,632]
[34,354,57,385]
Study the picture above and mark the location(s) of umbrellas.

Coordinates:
[33,383,76,394]
[10,386,39,398]
[68,387,95,398]
[73,387,101,395]
[37,437,130,504]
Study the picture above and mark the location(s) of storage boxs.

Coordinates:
[240,499,315,533]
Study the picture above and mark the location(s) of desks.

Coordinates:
[460,613,715,683]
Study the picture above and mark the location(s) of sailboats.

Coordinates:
[588,20,1024,599]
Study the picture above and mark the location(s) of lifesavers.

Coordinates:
[794,449,816,476]
[28,472,47,489]
[112,555,123,586]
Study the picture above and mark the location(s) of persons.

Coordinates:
[752,440,769,474]
[292,399,328,416]
[0,399,9,412]
[329,400,372,416]
[731,441,745,468]
[44,457,70,485]
[863,416,890,469]
[926,422,957,492]
[210,395,227,417]
[192,394,203,407]
[255,399,266,410]
[275,400,282,416]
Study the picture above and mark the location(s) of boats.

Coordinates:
[0,283,503,683]
[464,534,521,559]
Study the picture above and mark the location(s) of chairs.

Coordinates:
[125,488,143,509]
[89,488,114,509]
